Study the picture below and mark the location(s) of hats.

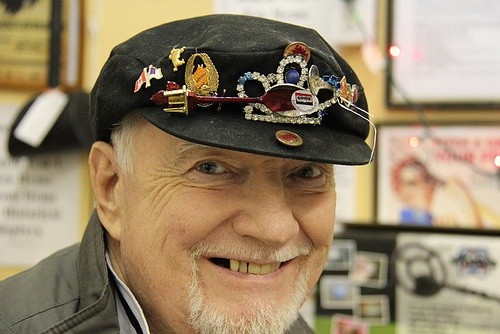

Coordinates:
[87,14,377,166]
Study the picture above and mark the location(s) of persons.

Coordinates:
[0,15,373,334]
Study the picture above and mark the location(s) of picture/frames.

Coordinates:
[374,120,500,234]
[384,0,500,108]
